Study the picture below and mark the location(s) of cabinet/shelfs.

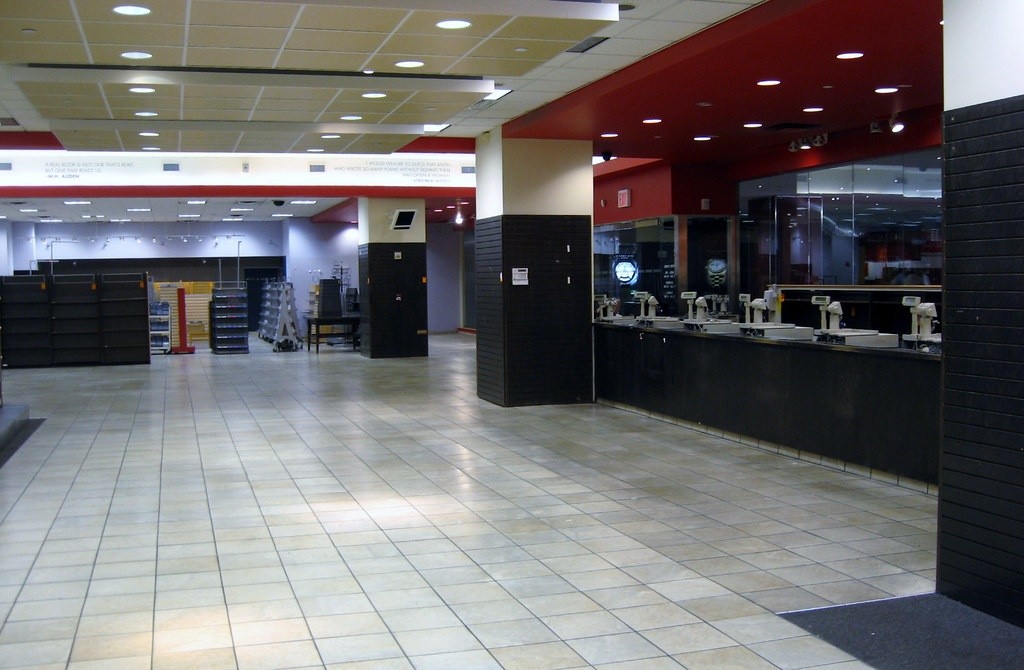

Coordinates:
[0,275,46,370]
[97,273,151,365]
[209,288,250,355]
[148,301,173,355]
[46,274,97,368]
[257,277,304,353]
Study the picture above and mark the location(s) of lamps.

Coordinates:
[868,116,905,134]
[789,133,827,152]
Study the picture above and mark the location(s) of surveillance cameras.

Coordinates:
[273,201,285,206]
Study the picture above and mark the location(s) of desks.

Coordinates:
[307,317,360,354]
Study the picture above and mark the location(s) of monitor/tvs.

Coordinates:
[390,210,417,231]
